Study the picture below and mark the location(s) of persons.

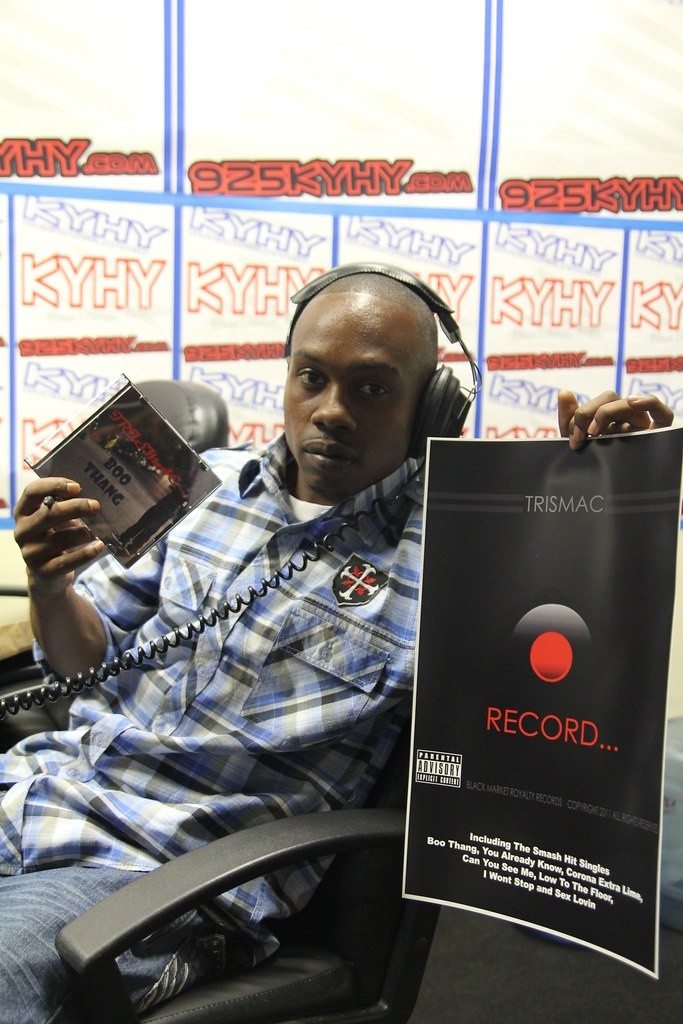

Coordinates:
[0,264,673,1024]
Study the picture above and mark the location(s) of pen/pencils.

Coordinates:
[43,496,55,507]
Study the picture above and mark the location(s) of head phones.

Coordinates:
[284,262,483,462]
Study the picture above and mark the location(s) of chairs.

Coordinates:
[133,381,228,453]
[0,665,444,1023]
